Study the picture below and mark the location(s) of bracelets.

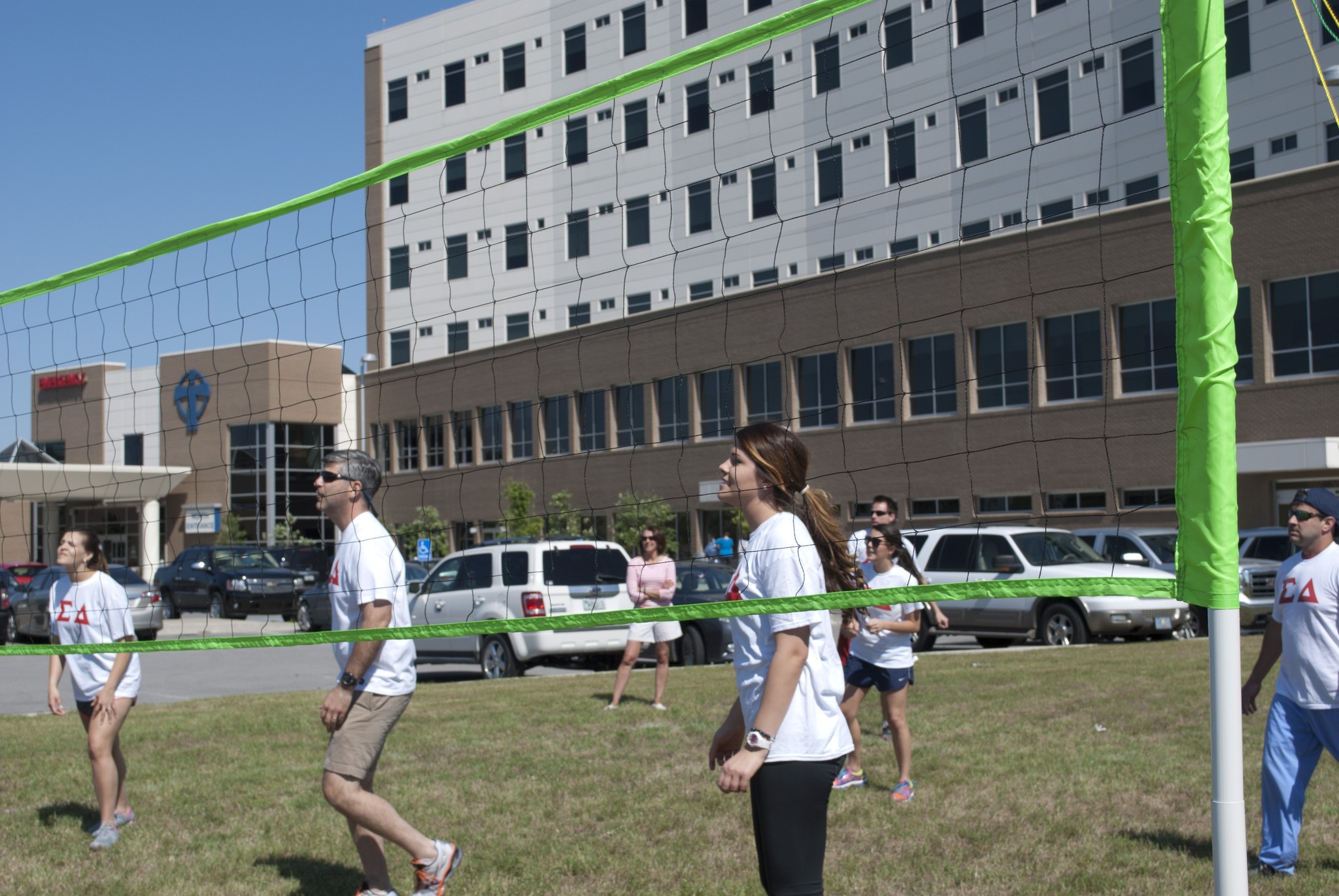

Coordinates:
[750,727,776,742]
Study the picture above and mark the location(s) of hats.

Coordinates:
[1293,487,1339,526]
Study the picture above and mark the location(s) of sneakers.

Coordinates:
[830,764,868,788]
[410,838,462,896]
[92,805,136,836]
[891,779,914,803]
[352,879,399,896]
[89,823,120,851]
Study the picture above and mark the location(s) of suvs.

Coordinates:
[898,525,1192,652]
[405,539,638,683]
[1067,527,1285,643]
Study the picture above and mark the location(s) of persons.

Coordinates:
[1240,483,1339,882]
[831,494,950,800]
[603,526,676,713]
[48,525,142,847]
[709,423,869,896]
[313,450,462,896]
[703,531,737,563]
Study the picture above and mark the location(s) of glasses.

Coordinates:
[871,509,890,516]
[1287,509,1324,522]
[319,471,357,483]
[864,535,888,547]
[642,536,656,541]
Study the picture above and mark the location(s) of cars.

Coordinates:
[265,547,334,580]
[293,562,426,633]
[152,546,322,621]
[625,560,740,666]
[1237,527,1303,561]
[0,567,21,645]
[7,562,165,643]
[0,561,49,586]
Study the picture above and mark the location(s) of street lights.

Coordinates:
[358,352,378,453]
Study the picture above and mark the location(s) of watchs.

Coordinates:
[746,731,772,750]
[338,672,365,689]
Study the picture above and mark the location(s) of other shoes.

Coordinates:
[603,705,619,710]
[882,725,892,741]
[651,703,666,711]
[1248,859,1291,880]
